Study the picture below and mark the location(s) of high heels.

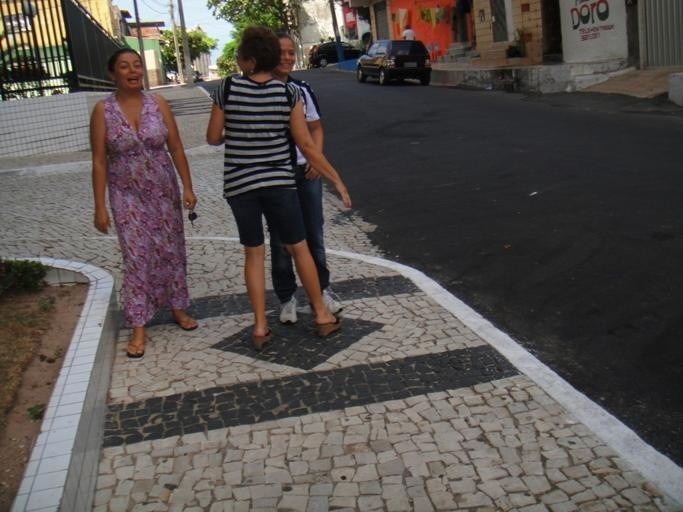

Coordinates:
[253,326,272,349]
[315,316,343,336]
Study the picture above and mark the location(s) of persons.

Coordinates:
[205,23,353,351]
[268,33,345,327]
[400,24,415,41]
[86,47,200,360]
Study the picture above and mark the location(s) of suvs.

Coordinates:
[355,39,432,86]
[308,40,361,69]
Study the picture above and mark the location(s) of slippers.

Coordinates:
[172,311,198,330]
[126,340,145,358]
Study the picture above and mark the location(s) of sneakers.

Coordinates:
[309,288,343,314]
[279,296,298,324]
